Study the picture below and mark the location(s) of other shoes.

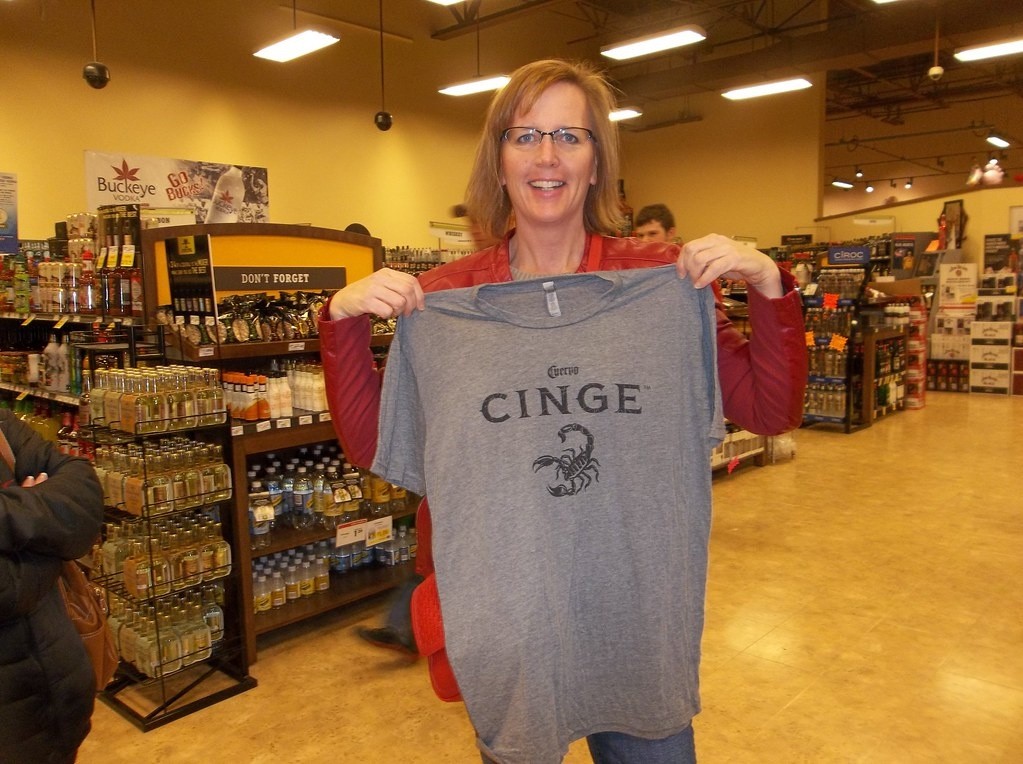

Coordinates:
[358,628,419,656]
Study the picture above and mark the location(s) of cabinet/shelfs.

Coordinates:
[55,409,258,734]
[0,310,143,406]
[801,263,877,433]
[139,223,425,669]
[859,294,912,424]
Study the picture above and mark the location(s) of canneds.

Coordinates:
[37,238,98,313]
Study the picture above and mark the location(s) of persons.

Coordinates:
[316,59,809,764]
[0,406,104,764]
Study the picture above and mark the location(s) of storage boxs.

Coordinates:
[928,263,1023,361]
[906,306,926,410]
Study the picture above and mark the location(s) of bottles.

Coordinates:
[204,165,244,223]
[789,232,890,299]
[804,307,905,418]
[1,253,418,678]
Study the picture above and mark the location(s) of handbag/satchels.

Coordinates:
[0,429,119,698]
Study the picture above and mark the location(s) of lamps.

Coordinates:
[954,0,1023,62]
[833,168,914,193]
[437,7,512,97]
[607,99,643,122]
[718,0,816,102]
[985,130,1012,148]
[251,0,341,64]
[599,0,707,61]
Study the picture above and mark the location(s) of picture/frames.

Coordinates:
[943,199,963,250]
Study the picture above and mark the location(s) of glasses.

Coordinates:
[500,127,597,152]
